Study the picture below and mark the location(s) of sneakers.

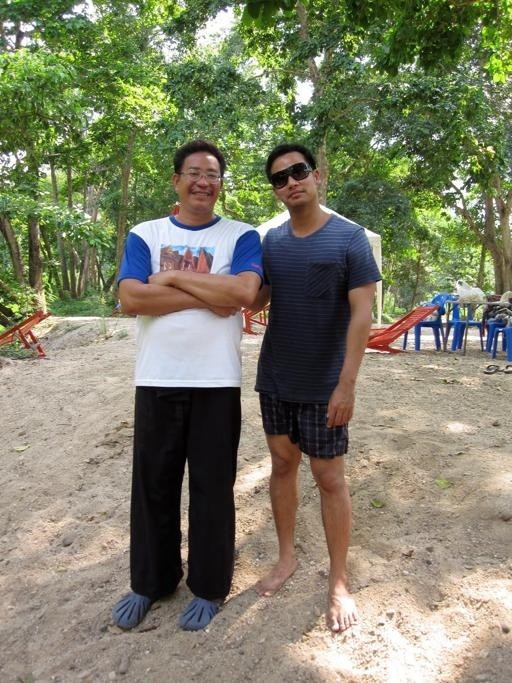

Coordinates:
[112,592,151,629]
[178,597,219,630]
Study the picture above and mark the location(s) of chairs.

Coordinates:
[406,290,512,362]
[364,302,439,356]
[0,307,51,360]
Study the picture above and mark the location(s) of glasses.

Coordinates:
[180,170,223,184]
[271,163,312,189]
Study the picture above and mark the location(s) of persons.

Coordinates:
[112,139,265,629]
[254,143,384,636]
